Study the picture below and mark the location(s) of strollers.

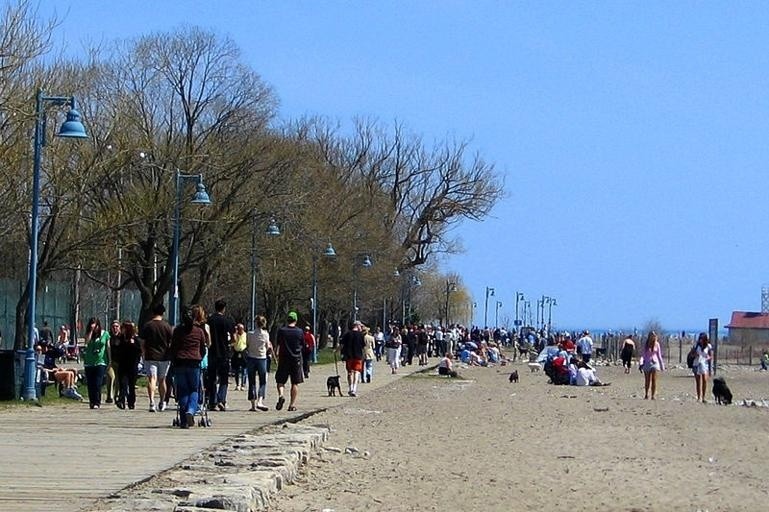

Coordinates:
[170,365,217,428]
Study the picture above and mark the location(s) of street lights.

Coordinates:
[380,269,559,338]
[18,85,90,404]
[166,168,211,329]
[246,208,280,337]
[347,252,370,319]
[306,236,334,364]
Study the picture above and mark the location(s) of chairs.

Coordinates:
[42,367,63,398]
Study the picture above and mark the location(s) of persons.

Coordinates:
[35,300,318,429]
[329,319,611,397]
[620,335,635,374]
[639,331,665,400]
[692,332,714,402]
[759,351,769,371]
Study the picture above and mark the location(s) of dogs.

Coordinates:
[712,376,732,405]
[509,370,518,382]
[518,346,528,357]
[327,376,343,396]
[53,366,76,390]
